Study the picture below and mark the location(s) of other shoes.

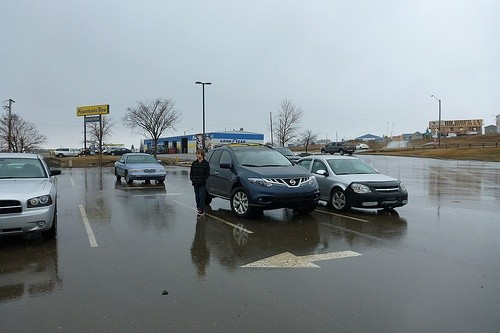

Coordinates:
[197,208,204,216]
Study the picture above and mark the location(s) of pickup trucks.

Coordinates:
[320,141,354,156]
[53,147,81,158]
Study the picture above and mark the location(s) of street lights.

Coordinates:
[430,94,441,148]
[270,112,282,147]
[195,81,212,153]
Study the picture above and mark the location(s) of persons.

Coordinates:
[190,149,210,215]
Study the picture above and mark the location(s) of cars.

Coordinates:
[294,154,409,213]
[88,144,132,156]
[0,152,62,239]
[355,143,370,150]
[114,152,167,185]
[202,142,321,220]
[270,146,301,164]
